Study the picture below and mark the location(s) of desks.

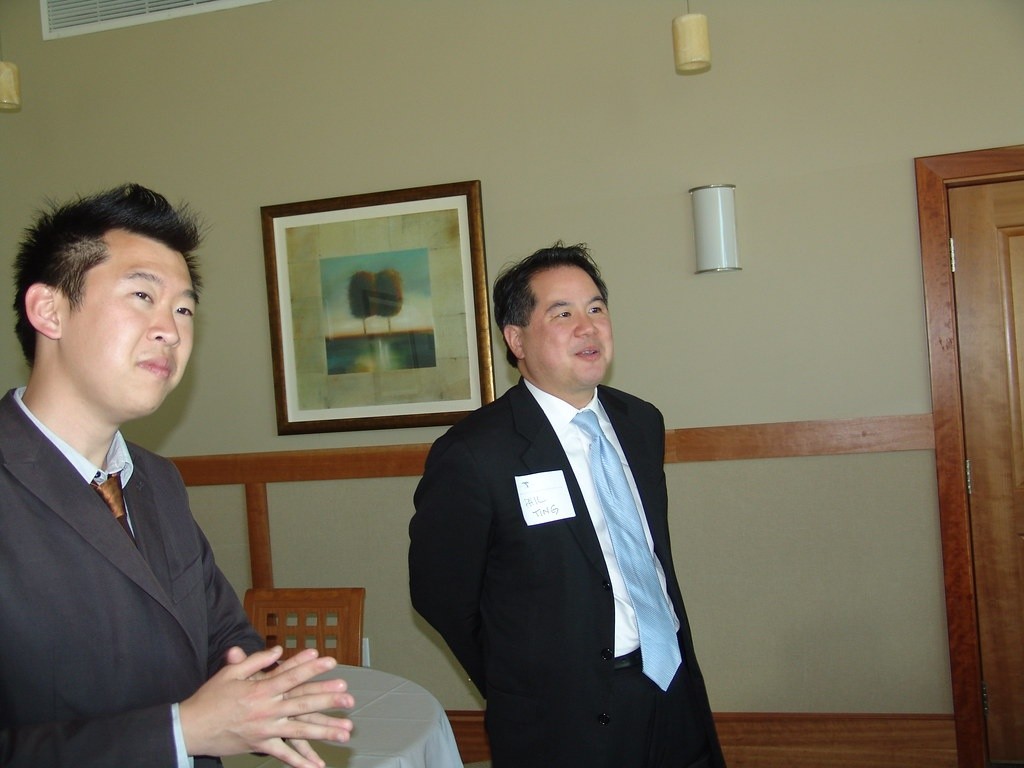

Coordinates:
[221,659,467,768]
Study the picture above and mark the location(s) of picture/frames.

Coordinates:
[258,179,497,438]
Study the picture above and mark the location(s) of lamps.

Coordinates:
[687,182,745,276]
[671,13,712,71]
[0,60,23,109]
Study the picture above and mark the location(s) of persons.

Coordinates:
[407,244,727,767]
[0,185,353,767]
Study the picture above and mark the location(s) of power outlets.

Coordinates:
[362,638,371,667]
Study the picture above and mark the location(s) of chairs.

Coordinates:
[243,586,365,668]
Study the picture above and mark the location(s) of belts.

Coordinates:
[614,648,642,669]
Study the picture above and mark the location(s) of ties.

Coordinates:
[90,471,137,548]
[571,408,682,691]
